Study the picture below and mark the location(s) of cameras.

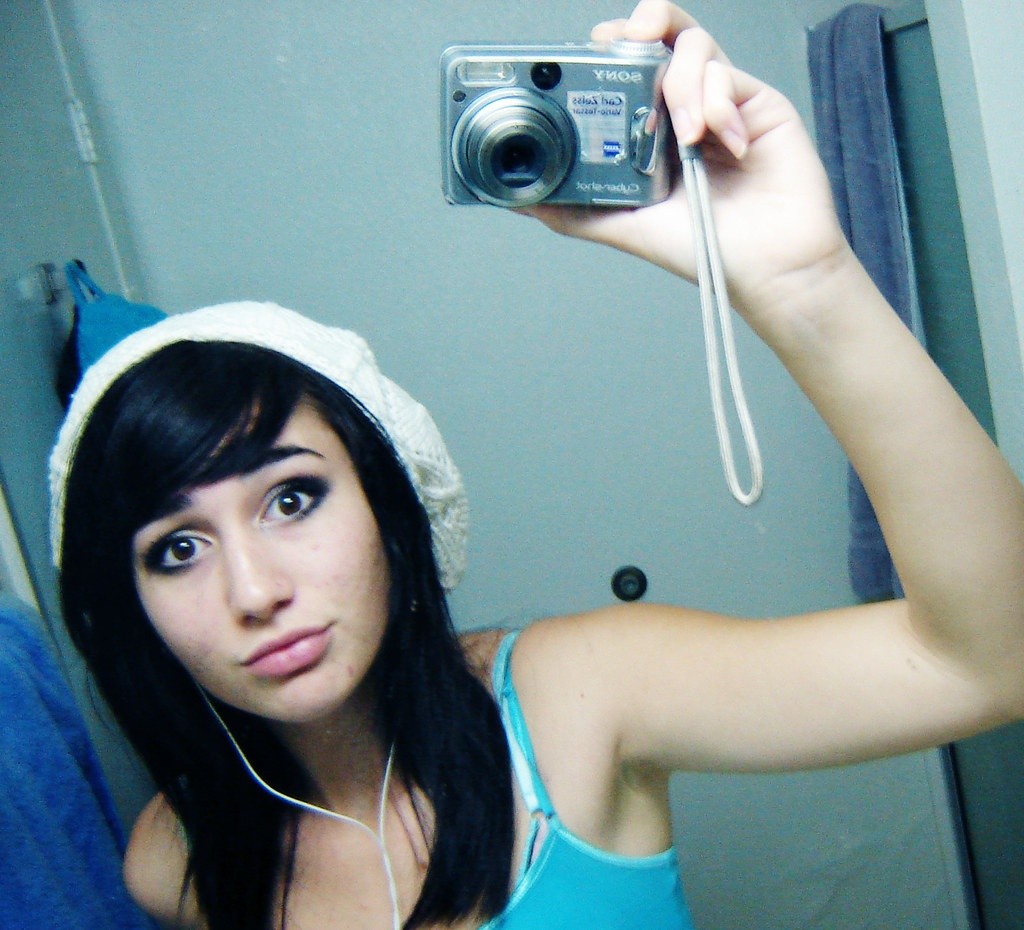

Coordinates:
[436,39,673,212]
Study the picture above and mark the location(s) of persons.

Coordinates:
[46,1,1024,930]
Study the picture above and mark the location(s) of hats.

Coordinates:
[48,302,471,592]
